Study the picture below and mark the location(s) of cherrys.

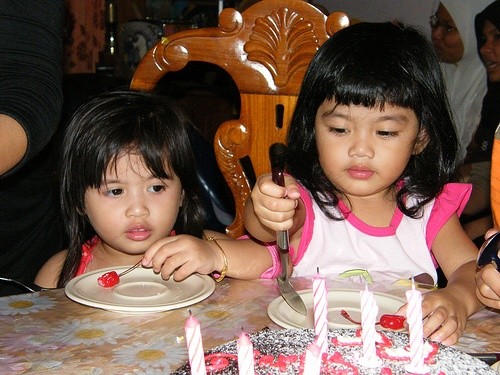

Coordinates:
[98,258,142,287]
[340,310,405,330]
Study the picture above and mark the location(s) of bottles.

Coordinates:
[103,0,120,66]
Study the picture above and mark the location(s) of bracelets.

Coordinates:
[208,237,228,283]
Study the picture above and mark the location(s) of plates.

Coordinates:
[65,265,216,313]
[268,290,408,335]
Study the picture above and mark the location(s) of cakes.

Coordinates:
[166,328,500,375]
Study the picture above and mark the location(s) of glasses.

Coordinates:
[429,16,458,33]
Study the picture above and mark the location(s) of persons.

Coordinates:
[35,88,283,289]
[240,19,487,348]
[0,0,73,298]
[427,0,500,309]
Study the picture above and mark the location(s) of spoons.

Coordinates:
[269,143,311,312]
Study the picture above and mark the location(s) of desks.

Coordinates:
[0,278,500,375]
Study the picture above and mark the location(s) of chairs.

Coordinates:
[131,1,351,239]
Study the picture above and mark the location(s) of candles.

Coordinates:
[360,282,377,368]
[312,267,328,352]
[406,277,423,369]
[304,343,321,375]
[236,327,254,375]
[184,309,206,375]
